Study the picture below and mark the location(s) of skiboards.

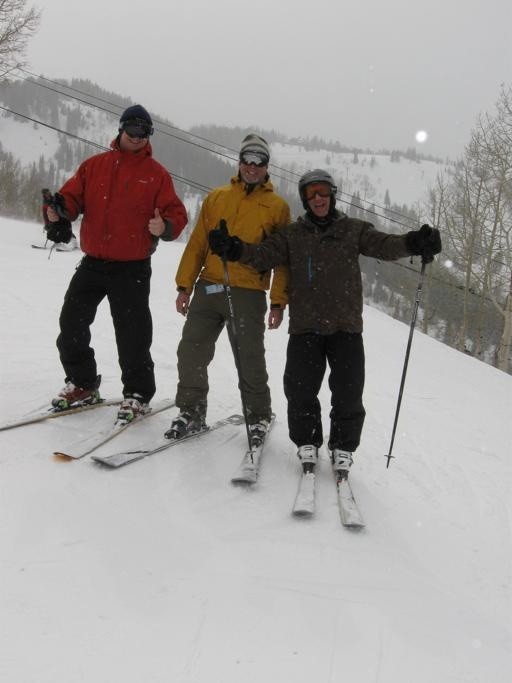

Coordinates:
[293,434,365,527]
[94,414,276,482]
[1,395,176,458]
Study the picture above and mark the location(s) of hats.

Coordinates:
[120,105,152,127]
[239,134,269,157]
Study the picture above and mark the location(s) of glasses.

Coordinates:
[240,152,269,166]
[124,121,151,138]
[300,181,332,200]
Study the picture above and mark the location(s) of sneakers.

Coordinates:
[117,394,149,419]
[249,418,269,437]
[51,381,100,406]
[164,407,205,439]
[332,449,353,471]
[297,445,318,465]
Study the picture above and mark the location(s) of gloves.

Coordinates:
[405,224,441,255]
[41,189,71,243]
[208,219,243,261]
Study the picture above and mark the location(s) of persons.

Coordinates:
[207,166,444,475]
[165,131,295,449]
[45,101,189,428]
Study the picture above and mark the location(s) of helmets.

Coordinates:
[299,170,336,209]
